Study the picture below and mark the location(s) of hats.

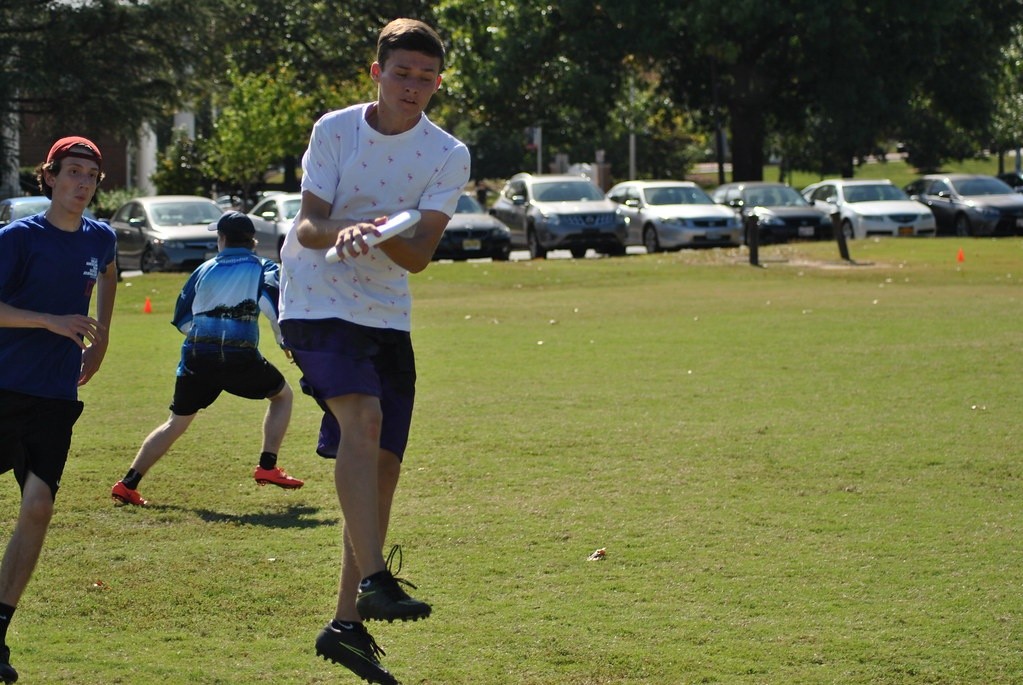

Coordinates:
[46,136,101,171]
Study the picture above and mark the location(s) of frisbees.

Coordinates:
[325,207,423,265]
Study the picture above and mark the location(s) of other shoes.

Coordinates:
[0,645,18,682]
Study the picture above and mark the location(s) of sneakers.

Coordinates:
[112,480,146,507]
[355,544,431,623]
[253,465,303,489]
[314,618,397,685]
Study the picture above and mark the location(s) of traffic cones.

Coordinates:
[144,297,153,313]
[956,248,964,263]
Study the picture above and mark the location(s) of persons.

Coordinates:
[0,135,119,683]
[276,19,471,685]
[111,211,305,505]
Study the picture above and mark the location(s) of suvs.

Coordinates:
[488,173,628,261]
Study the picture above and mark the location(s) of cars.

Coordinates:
[902,174,1023,238]
[250,192,288,206]
[248,195,301,263]
[708,182,837,243]
[996,172,1023,194]
[110,196,225,281]
[215,195,255,215]
[608,181,745,255]
[0,196,97,230]
[430,193,513,261]
[799,177,936,239]
